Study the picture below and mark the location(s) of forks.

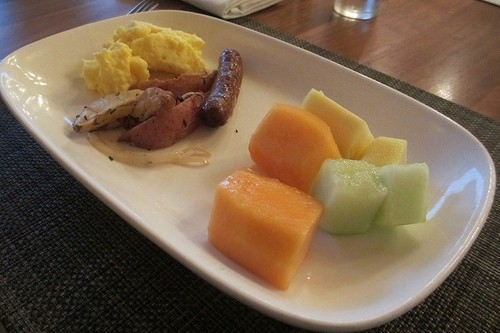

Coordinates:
[126,0,159,14]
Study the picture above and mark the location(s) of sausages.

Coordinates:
[200,48,243,128]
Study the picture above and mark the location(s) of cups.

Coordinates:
[333,0,382,21]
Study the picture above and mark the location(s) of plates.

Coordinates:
[0,10,497,333]
